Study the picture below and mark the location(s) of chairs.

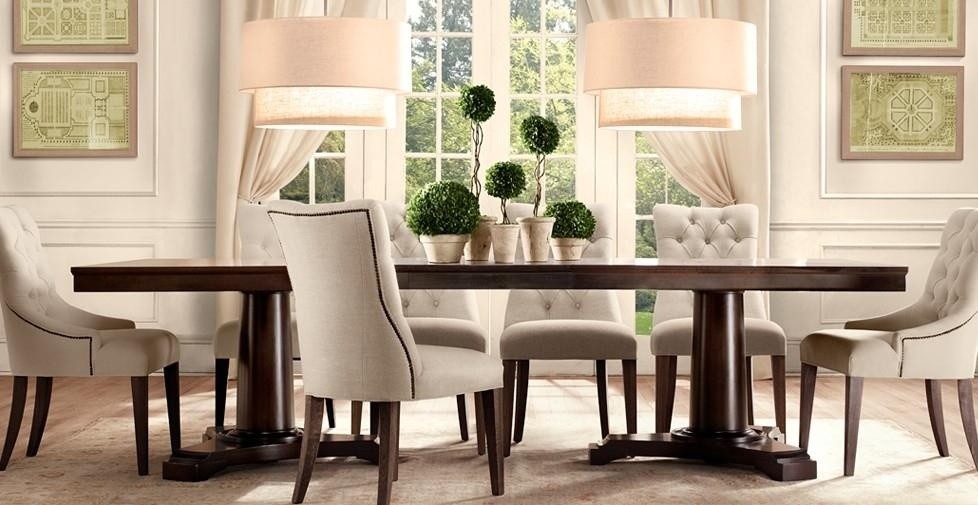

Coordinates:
[350,198,489,458]
[0,202,187,481]
[212,195,337,431]
[499,198,639,459]
[649,202,789,444]
[797,205,977,479]
[262,197,508,504]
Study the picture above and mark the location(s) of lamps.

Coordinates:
[235,0,407,132]
[581,0,761,132]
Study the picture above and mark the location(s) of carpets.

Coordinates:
[0,411,977,503]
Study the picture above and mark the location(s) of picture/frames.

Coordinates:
[11,60,141,159]
[840,0,968,57]
[839,63,965,162]
[9,0,140,54]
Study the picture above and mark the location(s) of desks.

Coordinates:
[68,257,911,486]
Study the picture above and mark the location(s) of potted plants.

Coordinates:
[543,200,598,262]
[404,179,483,265]
[481,160,528,264]
[454,81,498,264]
[515,114,561,263]
